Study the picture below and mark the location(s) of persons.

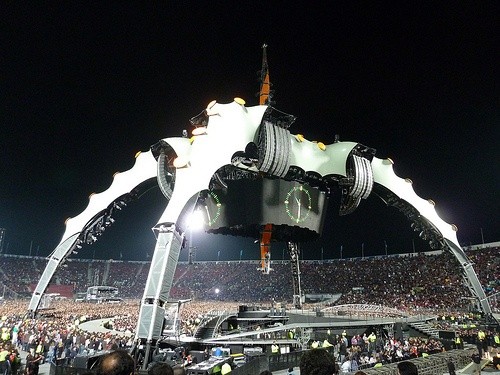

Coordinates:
[0,254,500,375]
